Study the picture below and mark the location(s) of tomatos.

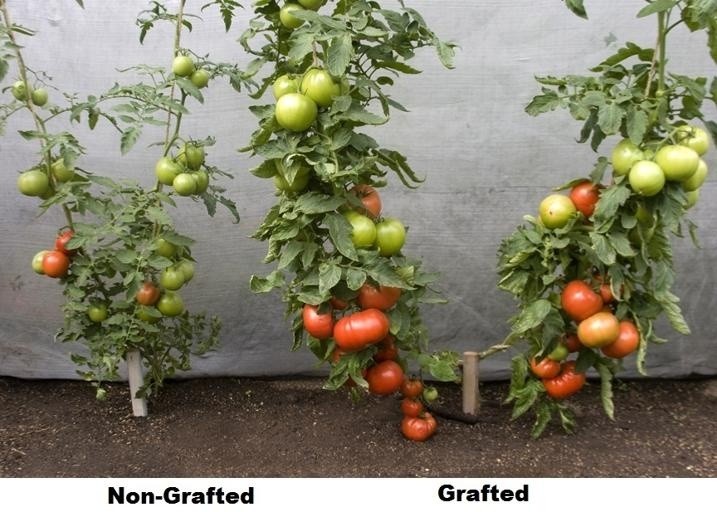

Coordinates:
[12,0,709,441]
